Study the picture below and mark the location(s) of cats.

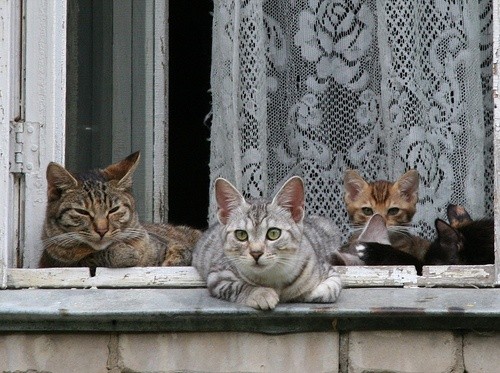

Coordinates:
[335,168,434,260]
[325,213,391,266]
[192,175,365,310]
[37,151,205,268]
[355,203,494,276]
